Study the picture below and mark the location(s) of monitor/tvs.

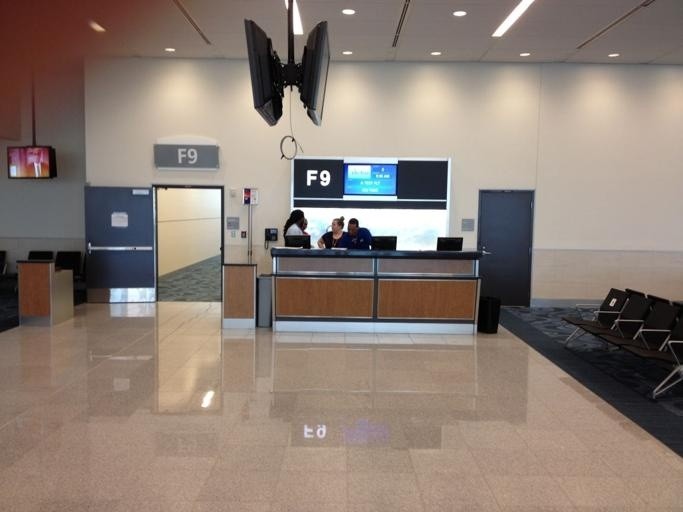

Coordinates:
[371,236,397,251]
[298,19,330,127]
[437,237,463,252]
[243,18,283,127]
[344,163,397,196]
[8,145,52,180]
[285,235,310,248]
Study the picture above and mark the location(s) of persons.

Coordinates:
[27,148,49,177]
[302,219,309,235]
[348,218,373,250]
[317,216,352,250]
[284,210,304,235]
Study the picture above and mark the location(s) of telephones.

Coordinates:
[263,227,278,250]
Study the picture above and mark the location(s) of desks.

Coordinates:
[273,246,481,336]
[16,260,74,327]
[223,263,256,330]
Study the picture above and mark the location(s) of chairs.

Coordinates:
[561,288,625,346]
[623,322,682,399]
[25,250,54,262]
[56,251,83,301]
[579,296,650,336]
[600,302,675,348]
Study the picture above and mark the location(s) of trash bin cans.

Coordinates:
[256,274,272,327]
[478,295,501,334]
